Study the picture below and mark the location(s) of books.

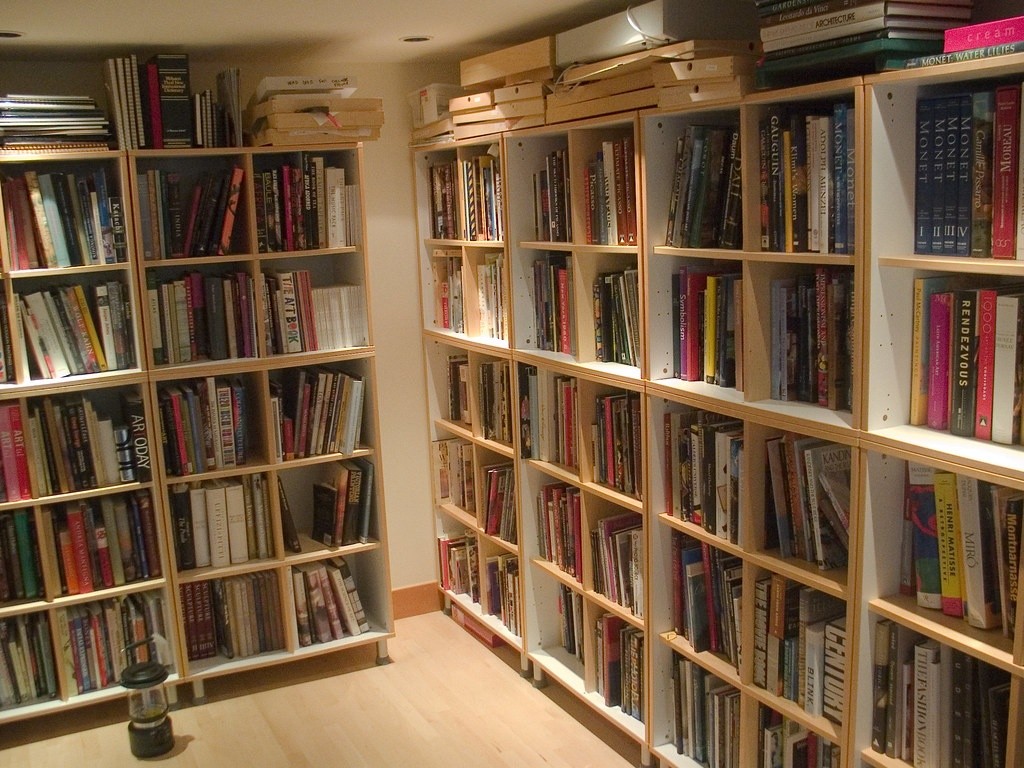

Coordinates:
[0,51,386,151]
[437,338,1021,666]
[0,459,376,610]
[0,366,367,513]
[434,426,1024,768]
[407,1,1024,159]
[0,155,363,277]
[422,87,1024,265]
[0,558,374,713]
[422,239,1023,456]
[0,261,370,386]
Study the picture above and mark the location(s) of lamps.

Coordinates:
[117,636,176,759]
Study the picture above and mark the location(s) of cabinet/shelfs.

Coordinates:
[125,136,400,703]
[407,50,1024,767]
[0,140,188,728]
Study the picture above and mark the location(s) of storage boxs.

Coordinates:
[450,600,509,647]
[247,1,763,145]
